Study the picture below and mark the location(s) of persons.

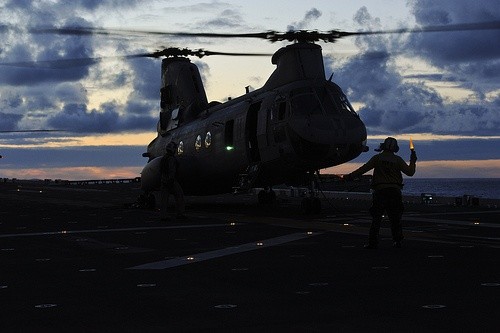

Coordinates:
[159,142,189,221]
[338,137,417,247]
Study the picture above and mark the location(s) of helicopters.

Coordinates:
[40,16,500,217]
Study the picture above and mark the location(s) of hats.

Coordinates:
[384,137,397,150]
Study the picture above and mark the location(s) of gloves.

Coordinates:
[411,151,417,160]
[344,173,352,177]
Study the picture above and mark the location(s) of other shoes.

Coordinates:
[392,240,406,250]
[361,241,378,248]
